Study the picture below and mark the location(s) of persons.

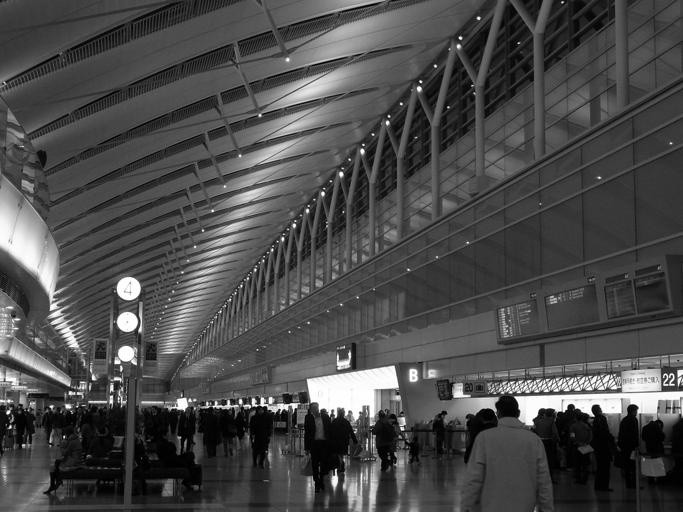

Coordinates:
[457,393,553,511]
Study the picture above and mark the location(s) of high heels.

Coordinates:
[43,481,64,495]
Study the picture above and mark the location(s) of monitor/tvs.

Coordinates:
[200,392,308,406]
[336,343,356,372]
[436,379,452,400]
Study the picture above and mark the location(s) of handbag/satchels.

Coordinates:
[641,457,666,477]
[300,453,314,477]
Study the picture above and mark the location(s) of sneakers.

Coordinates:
[315,464,345,494]
[380,457,443,474]
[570,480,647,493]
[181,440,269,469]
[0,442,60,456]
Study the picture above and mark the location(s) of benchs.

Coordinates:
[45,444,203,498]
